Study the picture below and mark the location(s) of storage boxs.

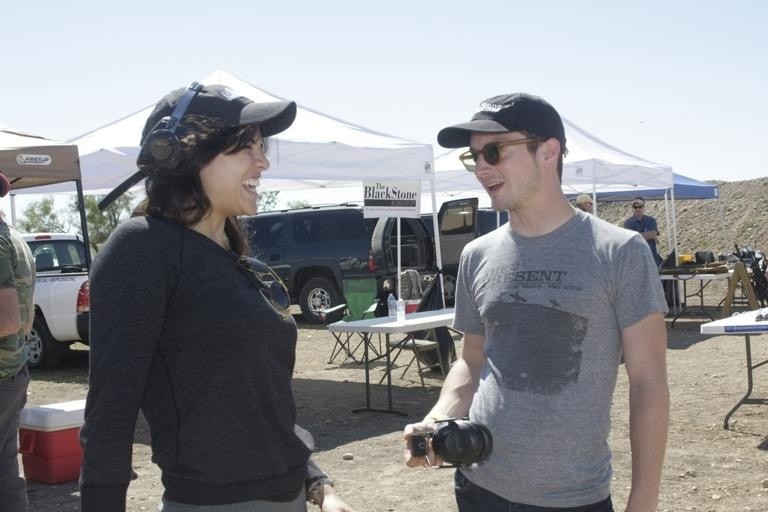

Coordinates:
[19,398,86,485]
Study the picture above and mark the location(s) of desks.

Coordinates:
[330,309,456,417]
[660,269,754,328]
[700,307,768,429]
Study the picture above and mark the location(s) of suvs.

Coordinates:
[235,197,478,325]
[416,208,508,304]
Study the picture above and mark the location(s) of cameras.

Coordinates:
[410,418,491,469]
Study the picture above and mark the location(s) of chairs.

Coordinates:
[379,329,446,388]
[327,299,382,366]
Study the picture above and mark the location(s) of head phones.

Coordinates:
[136,80,204,179]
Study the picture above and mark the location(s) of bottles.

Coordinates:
[752,247,762,270]
[386,293,396,317]
[396,297,406,325]
[704,249,712,268]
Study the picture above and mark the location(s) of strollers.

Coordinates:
[748,252,767,308]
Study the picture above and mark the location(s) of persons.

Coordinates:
[403,92,670,512]
[79,81,353,512]
[624,197,663,267]
[574,194,592,212]
[0,172,36,511]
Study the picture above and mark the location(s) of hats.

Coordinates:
[142,86,296,148]
[438,93,565,154]
[576,194,594,204]
[631,198,644,205]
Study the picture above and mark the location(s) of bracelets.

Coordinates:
[310,479,334,505]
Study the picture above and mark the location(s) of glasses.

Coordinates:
[459,138,546,172]
[634,206,642,209]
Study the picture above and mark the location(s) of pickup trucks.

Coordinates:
[11,232,92,369]
